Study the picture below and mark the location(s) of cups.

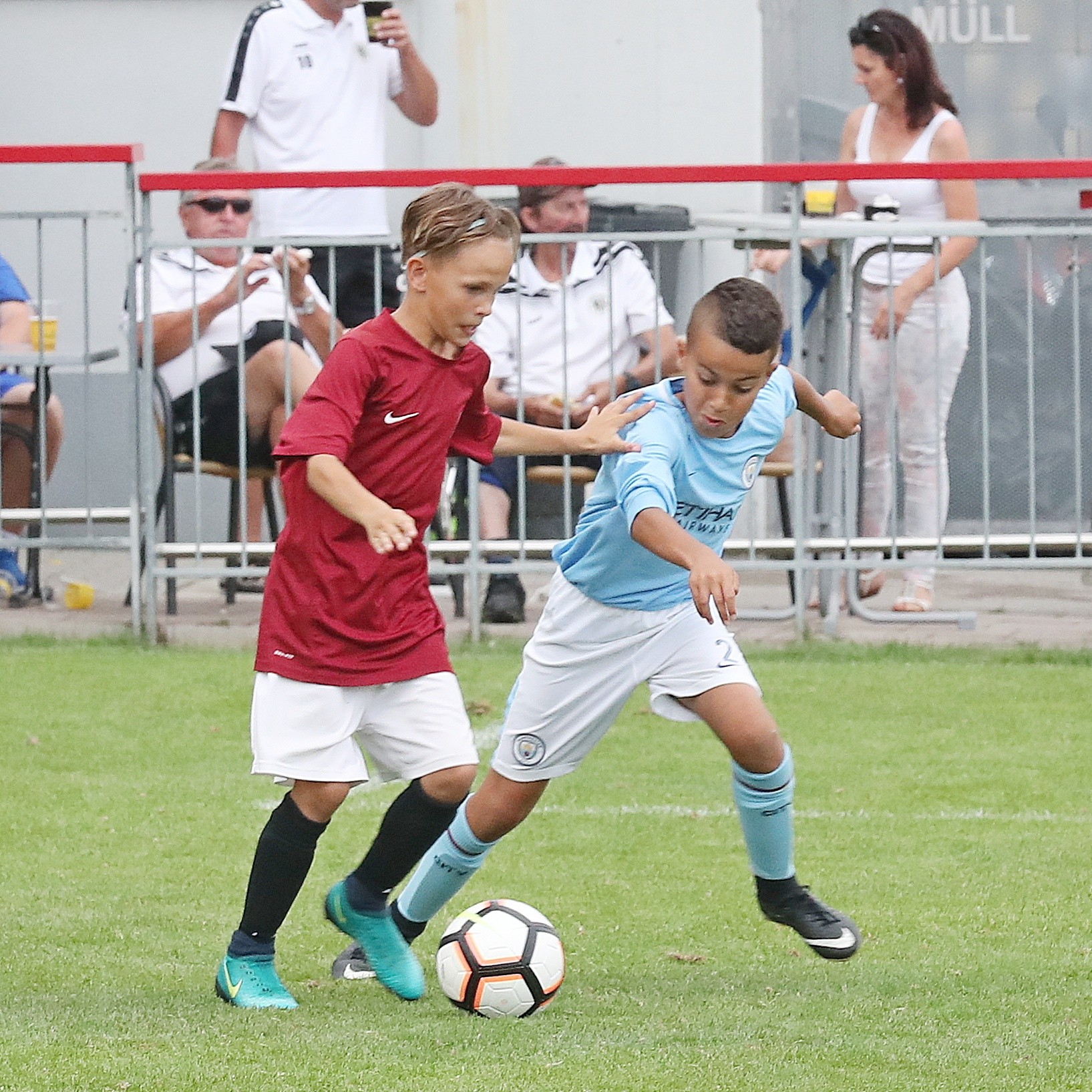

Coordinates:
[0,570,19,608]
[27,298,56,353]
[42,572,93,611]
[803,181,839,215]
[363,1,392,44]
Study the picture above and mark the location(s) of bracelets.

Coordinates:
[623,370,640,390]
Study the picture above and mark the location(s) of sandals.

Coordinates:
[806,569,883,612]
[894,582,933,612]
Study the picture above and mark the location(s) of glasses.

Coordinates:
[858,17,900,50]
[184,197,251,214]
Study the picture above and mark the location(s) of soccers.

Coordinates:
[435,898,566,1019]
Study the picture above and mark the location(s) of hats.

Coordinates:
[517,157,597,206]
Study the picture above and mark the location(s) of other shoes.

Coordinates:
[482,575,526,624]
[220,557,272,593]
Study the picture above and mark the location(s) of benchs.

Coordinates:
[456,457,822,615]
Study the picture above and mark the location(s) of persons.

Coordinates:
[123,156,345,582]
[215,177,655,1012]
[210,0,439,334]
[0,257,64,601]
[475,155,680,623]
[750,9,981,612]
[330,277,863,981]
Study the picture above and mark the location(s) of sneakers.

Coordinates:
[758,885,860,962]
[332,905,416,979]
[324,880,425,1000]
[0,563,54,608]
[215,954,299,1009]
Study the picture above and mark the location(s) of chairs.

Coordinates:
[127,356,280,614]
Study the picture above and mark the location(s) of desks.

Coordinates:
[0,348,119,607]
